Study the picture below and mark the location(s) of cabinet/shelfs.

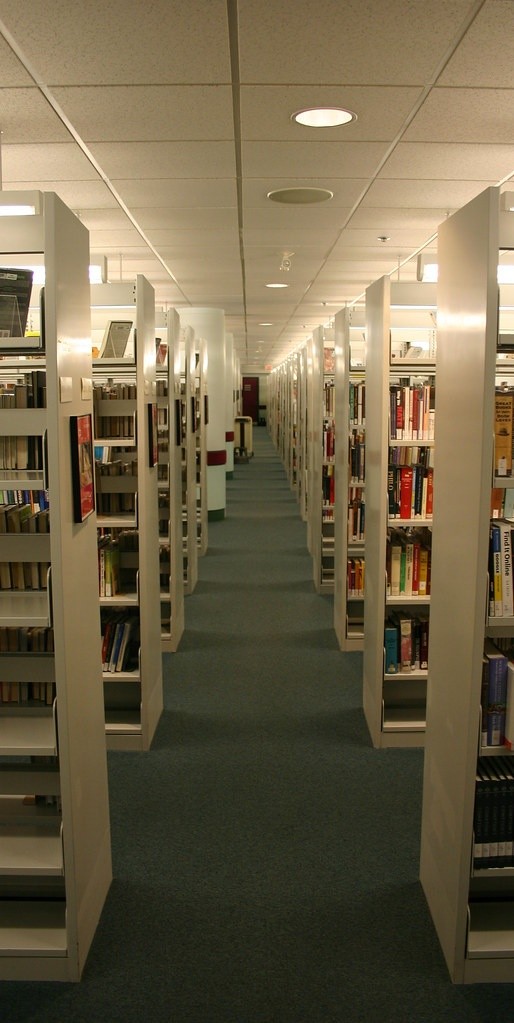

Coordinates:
[0,186,514,986]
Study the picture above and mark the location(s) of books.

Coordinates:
[270,382,514,895]
[0,380,203,813]
